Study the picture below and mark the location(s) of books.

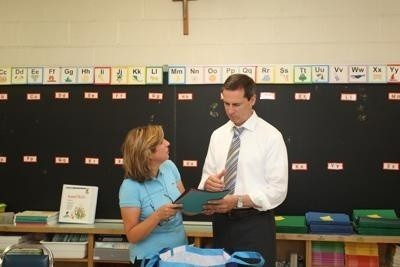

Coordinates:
[0,207,129,260]
[274,209,400,267]
[173,187,230,215]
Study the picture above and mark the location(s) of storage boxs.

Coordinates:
[39,240,88,259]
[0,235,29,250]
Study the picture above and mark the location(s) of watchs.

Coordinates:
[237,195,244,208]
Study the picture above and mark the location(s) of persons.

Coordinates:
[197,74,288,267]
[118,125,199,267]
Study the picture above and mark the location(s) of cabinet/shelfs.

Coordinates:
[0,222,400,267]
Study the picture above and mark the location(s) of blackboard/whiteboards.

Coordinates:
[1,82,400,227]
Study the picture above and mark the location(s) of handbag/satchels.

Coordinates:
[140,241,264,267]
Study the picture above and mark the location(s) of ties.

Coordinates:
[224,126,243,195]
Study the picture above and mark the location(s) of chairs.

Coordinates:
[2,254,49,267]
